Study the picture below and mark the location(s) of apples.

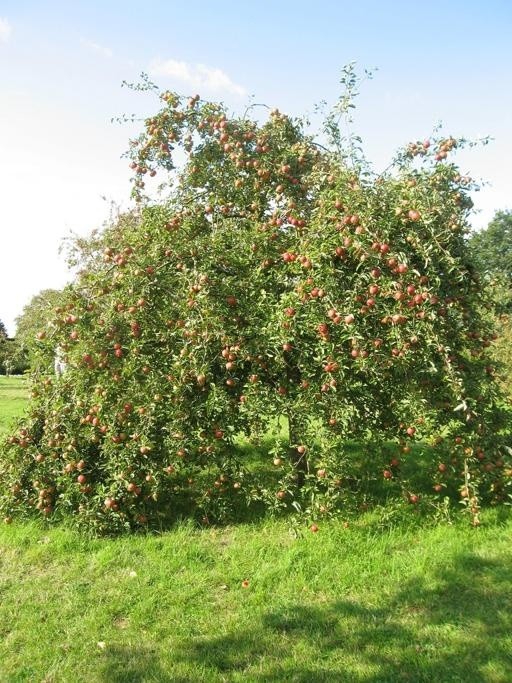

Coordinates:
[0,91,512,533]
[241,581,249,588]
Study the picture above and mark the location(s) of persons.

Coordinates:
[53,345,66,379]
